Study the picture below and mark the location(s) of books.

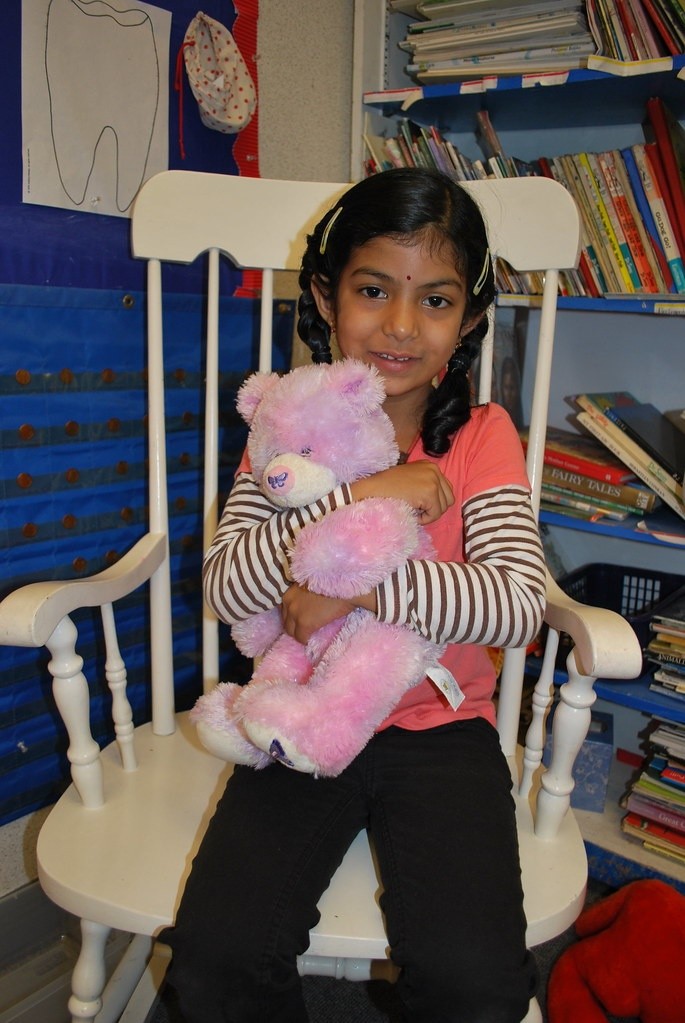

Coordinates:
[516,391,685,524]
[620,714,685,866]
[362,0,685,298]
[643,585,685,701]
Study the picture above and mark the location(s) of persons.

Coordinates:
[172,166,543,1023]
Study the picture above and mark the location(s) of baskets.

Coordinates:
[539,562,685,656]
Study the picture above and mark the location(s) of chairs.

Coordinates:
[0,169,643,1023]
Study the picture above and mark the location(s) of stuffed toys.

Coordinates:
[187,355,449,778]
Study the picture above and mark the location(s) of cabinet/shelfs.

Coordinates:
[348,0,685,893]
